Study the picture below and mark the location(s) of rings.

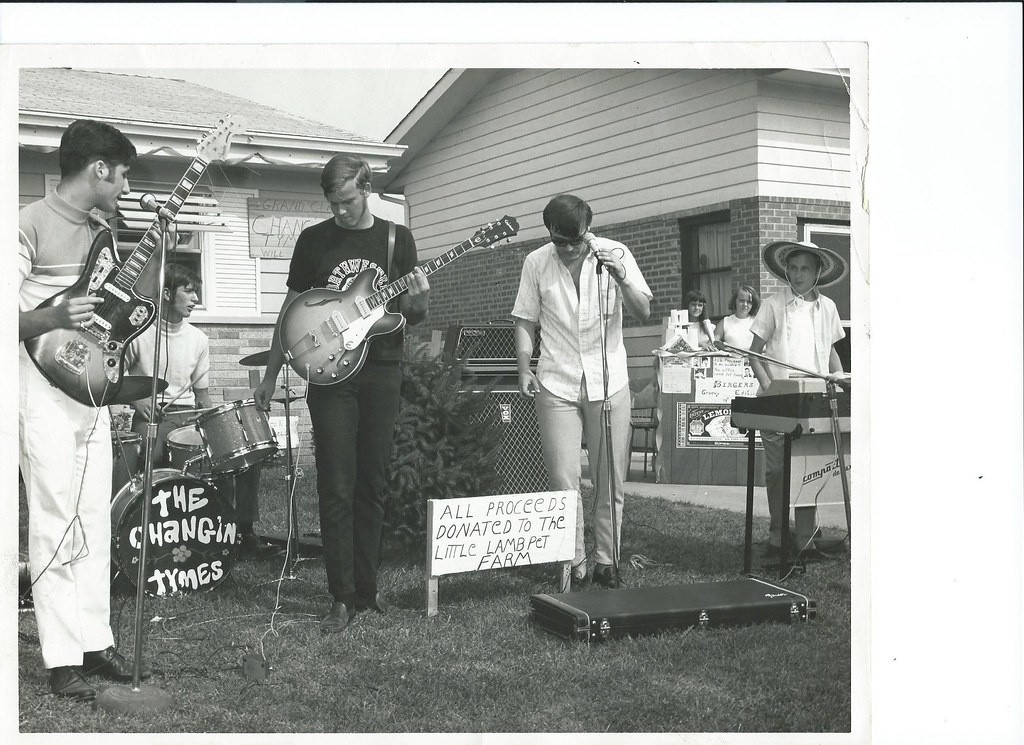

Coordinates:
[80,320,84,331]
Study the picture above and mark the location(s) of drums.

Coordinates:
[111,430,142,502]
[195,398,279,475]
[166,423,220,480]
[112,468,242,598]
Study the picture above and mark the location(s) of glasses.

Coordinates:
[550,227,587,247]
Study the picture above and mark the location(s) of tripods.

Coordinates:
[245,363,328,591]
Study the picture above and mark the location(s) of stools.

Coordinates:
[630,405,657,477]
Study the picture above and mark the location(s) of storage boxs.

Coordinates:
[528,576,818,641]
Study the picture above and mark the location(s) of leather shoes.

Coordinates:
[82,646,151,681]
[49,665,94,702]
[354,593,398,615]
[320,602,353,634]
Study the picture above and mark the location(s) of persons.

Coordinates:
[20,119,181,700]
[682,290,717,352]
[253,153,432,633]
[748,241,847,563]
[707,285,761,352]
[123,263,287,563]
[509,194,655,590]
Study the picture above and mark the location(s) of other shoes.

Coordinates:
[592,565,627,589]
[238,530,281,560]
[571,573,588,592]
[802,547,839,561]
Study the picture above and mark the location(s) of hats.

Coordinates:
[761,240,849,288]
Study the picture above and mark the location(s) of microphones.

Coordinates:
[138,191,179,224]
[581,231,619,274]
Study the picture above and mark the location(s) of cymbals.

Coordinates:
[271,397,305,403]
[107,376,169,405]
[239,350,286,366]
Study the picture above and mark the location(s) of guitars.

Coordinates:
[279,214,520,387]
[24,113,246,407]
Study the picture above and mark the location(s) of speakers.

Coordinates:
[441,319,549,494]
